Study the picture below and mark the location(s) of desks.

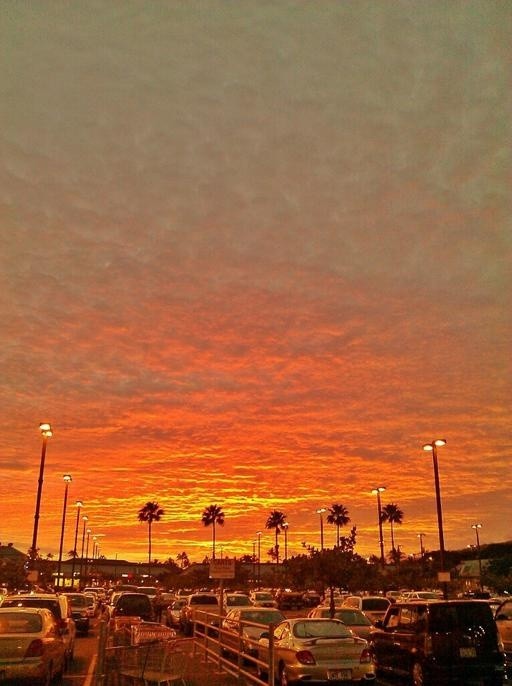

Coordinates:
[31,422,100,590]
[113,552,121,579]
[246,439,486,596]
[219,543,224,562]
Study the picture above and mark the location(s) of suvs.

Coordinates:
[372,599,509,683]
[2,593,82,665]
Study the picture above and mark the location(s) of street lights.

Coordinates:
[113,552,121,579]
[246,439,486,596]
[31,422,100,590]
[219,543,224,562]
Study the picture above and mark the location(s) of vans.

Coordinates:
[342,596,393,626]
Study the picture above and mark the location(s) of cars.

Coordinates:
[493,598,510,656]
[306,607,379,644]
[0,580,512,635]
[2,607,70,683]
[220,607,291,666]
[256,617,377,684]
